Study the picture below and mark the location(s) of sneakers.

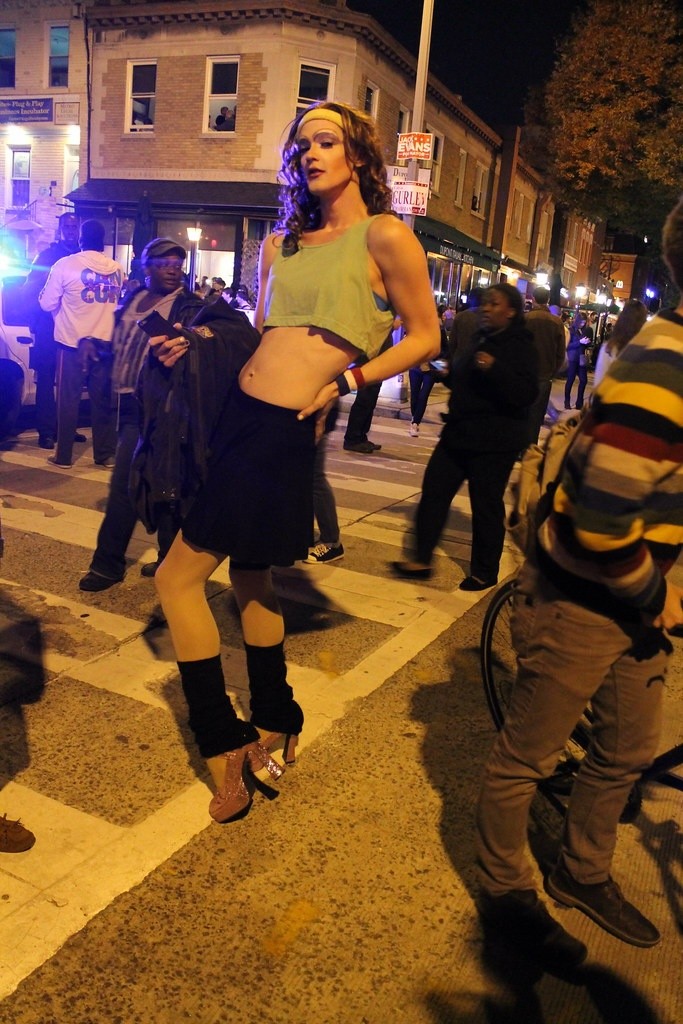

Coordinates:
[37,434,56,449]
[75,432,87,443]
[478,886,587,970]
[303,544,347,563]
[48,454,73,468]
[95,456,115,467]
[547,850,661,947]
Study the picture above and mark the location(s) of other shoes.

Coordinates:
[360,440,381,450]
[458,576,498,590]
[140,555,169,577]
[344,442,374,454]
[78,571,115,592]
[0,812,36,853]
[409,424,420,437]
[384,561,433,580]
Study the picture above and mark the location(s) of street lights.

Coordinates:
[187,220,204,292]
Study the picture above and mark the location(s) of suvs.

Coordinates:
[0,274,95,430]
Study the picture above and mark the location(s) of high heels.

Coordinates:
[242,732,297,771]
[207,740,285,823]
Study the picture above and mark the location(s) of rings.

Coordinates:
[478,360,485,364]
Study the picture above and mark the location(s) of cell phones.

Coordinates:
[137,310,181,347]
[427,360,444,371]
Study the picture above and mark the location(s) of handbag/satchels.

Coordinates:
[580,354,591,367]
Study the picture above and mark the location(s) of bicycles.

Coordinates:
[480,570,683,799]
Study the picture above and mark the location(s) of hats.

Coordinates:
[141,237,187,266]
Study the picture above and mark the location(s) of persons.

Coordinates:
[343,287,648,457]
[474,196,683,972]
[150,101,441,821]
[209,105,236,131]
[16,212,249,590]
[383,282,538,590]
[0,812,36,853]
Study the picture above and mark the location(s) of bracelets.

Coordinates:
[335,368,365,395]
[197,326,214,337]
